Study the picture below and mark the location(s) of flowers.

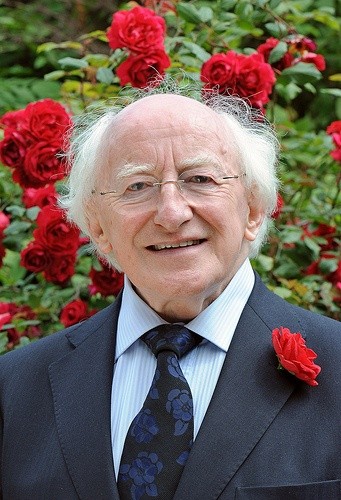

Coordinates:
[272,327,320,386]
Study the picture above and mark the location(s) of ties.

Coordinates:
[116,324,204,500]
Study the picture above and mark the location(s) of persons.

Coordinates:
[0,92,341,500]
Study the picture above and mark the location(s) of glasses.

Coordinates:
[91,166,247,203]
